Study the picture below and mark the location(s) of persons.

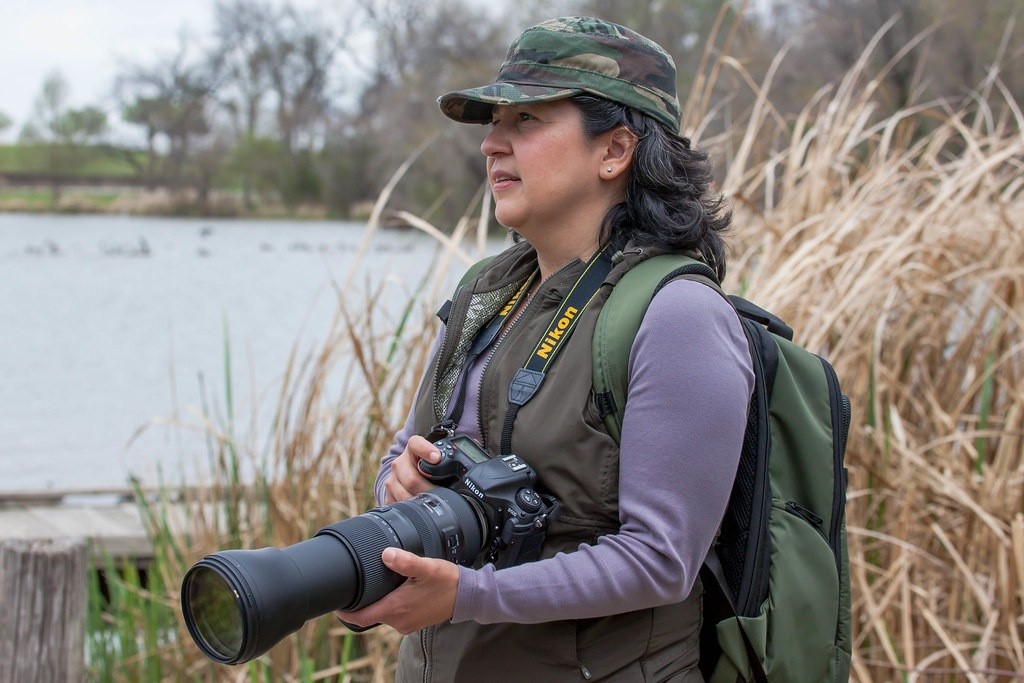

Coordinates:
[332,14,851,683]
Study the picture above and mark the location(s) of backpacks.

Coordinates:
[455,254,850,683]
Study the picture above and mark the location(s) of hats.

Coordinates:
[439,16,682,133]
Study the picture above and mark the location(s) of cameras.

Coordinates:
[181,433,554,666]
